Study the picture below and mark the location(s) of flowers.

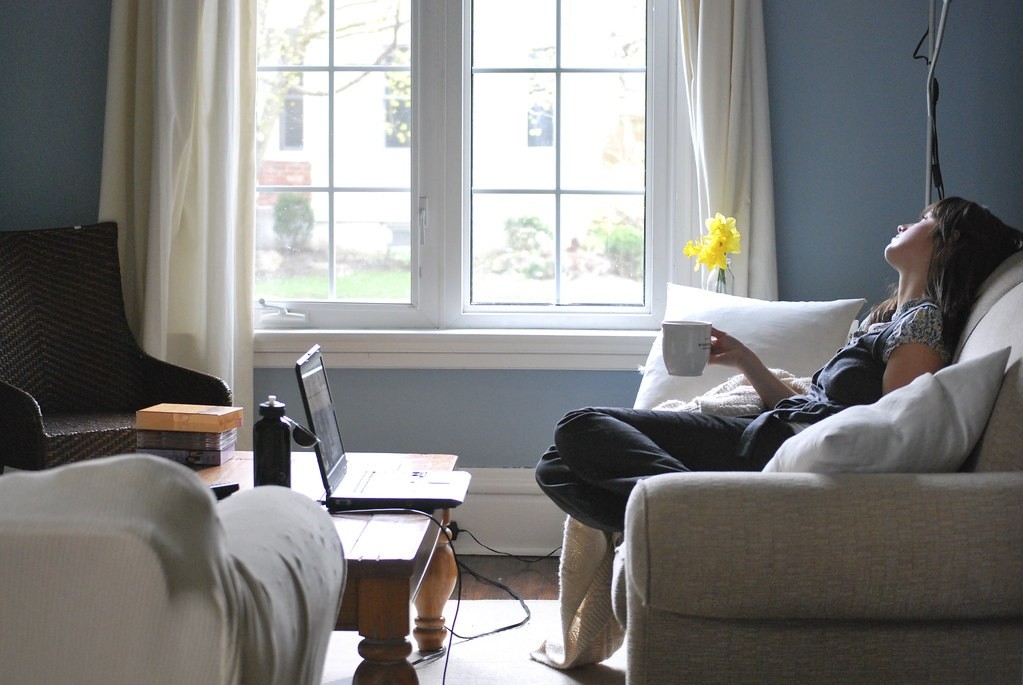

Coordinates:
[681,212,743,295]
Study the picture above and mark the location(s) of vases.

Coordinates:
[705,259,736,297]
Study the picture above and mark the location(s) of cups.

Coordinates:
[661,320,713,378]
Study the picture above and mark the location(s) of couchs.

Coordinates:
[608,242,1023,685]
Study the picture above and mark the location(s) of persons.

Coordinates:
[534,193,1023,531]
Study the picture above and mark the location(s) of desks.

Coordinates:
[192,450,461,685]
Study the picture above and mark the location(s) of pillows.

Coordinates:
[755,346,1013,474]
[632,281,868,412]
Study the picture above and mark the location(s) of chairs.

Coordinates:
[1,220,236,472]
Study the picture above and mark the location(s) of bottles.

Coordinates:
[252,395,324,491]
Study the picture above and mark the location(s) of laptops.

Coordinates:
[293,343,471,508]
